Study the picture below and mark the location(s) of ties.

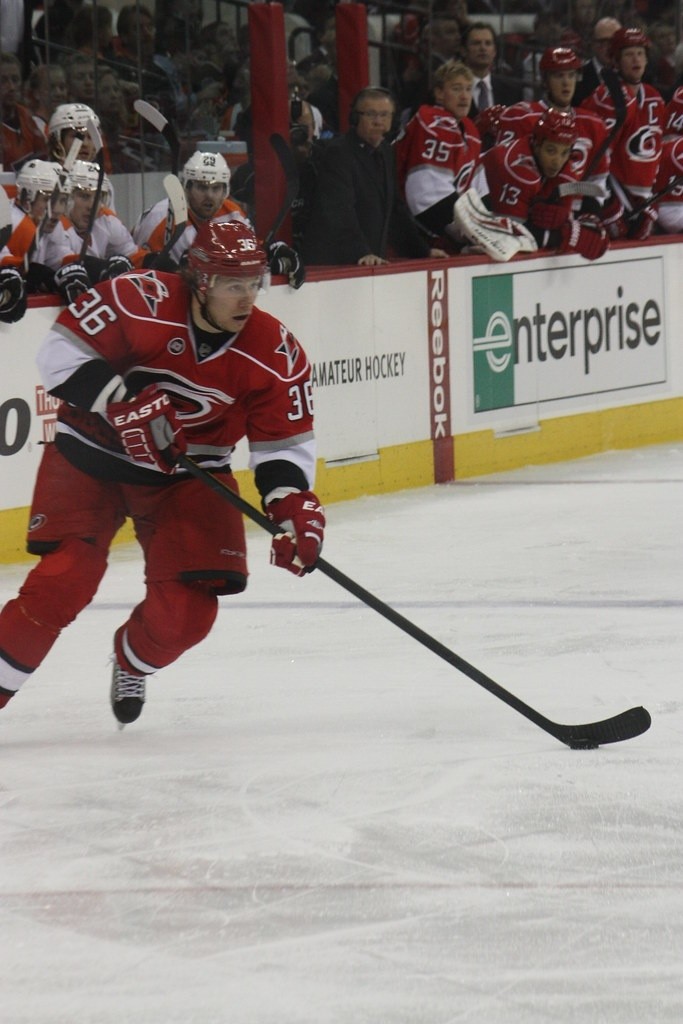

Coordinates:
[477,80,489,112]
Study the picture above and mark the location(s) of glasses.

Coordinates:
[358,108,394,122]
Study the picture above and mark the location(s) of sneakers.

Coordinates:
[105,625,147,731]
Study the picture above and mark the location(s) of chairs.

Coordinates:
[29,0,548,239]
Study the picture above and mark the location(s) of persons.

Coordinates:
[0,0,683,322]
[0,221,326,725]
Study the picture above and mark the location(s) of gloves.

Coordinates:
[106,385,187,476]
[530,202,569,230]
[556,217,610,261]
[600,196,632,240]
[98,254,133,283]
[0,264,28,323]
[266,490,326,577]
[26,261,58,294]
[266,236,307,290]
[54,263,93,308]
[634,199,660,241]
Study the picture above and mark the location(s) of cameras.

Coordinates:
[288,100,308,146]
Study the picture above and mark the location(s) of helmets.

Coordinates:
[48,102,101,143]
[66,159,108,194]
[181,150,232,199]
[15,159,72,202]
[187,218,270,276]
[540,47,584,84]
[533,106,580,148]
[608,27,652,58]
